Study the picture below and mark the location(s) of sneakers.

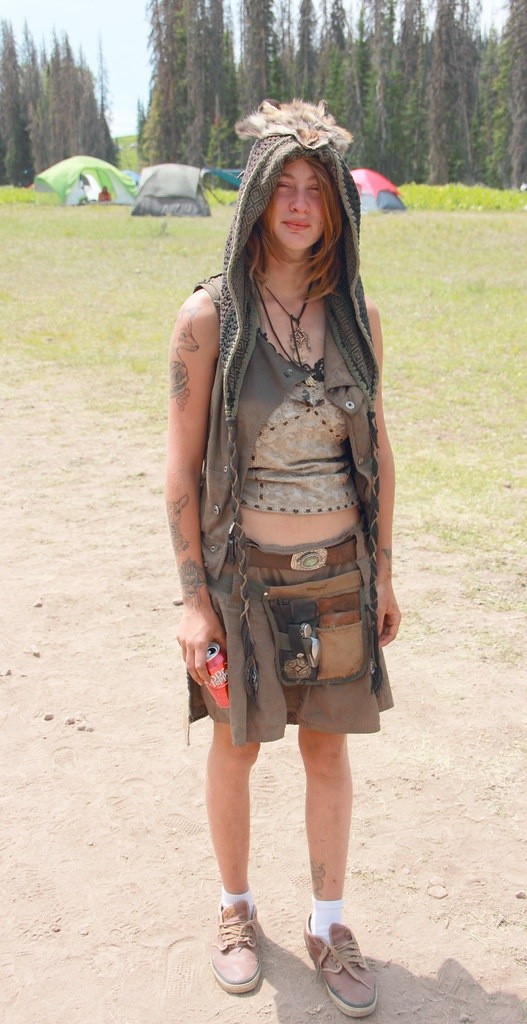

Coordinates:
[303,913,377,1016]
[210,899,260,993]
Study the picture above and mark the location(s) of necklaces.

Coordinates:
[250,274,317,388]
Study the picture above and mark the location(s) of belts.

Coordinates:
[244,539,357,571]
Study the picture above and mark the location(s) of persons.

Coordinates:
[79,187,88,204]
[166,99,401,1017]
[99,186,111,201]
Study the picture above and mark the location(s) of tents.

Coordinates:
[34,155,141,205]
[207,170,243,188]
[131,164,210,216]
[349,169,406,211]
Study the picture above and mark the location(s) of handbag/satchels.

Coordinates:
[263,589,368,686]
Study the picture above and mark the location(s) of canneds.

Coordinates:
[204,641,230,709]
